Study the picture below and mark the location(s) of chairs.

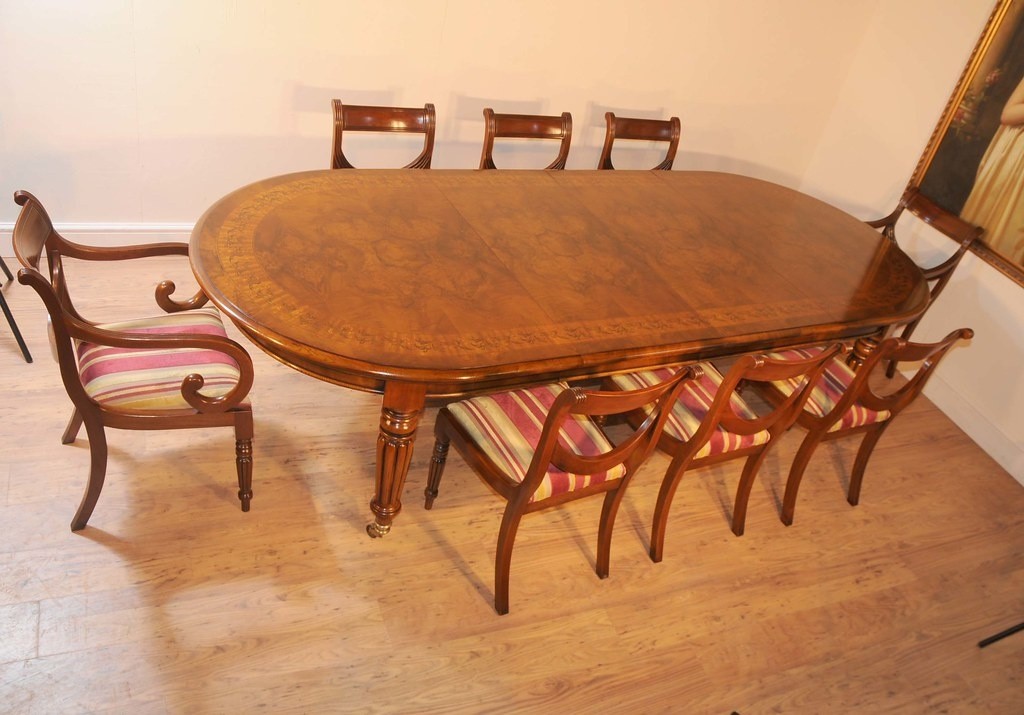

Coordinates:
[862,188,983,377]
[327,97,437,171]
[477,106,574,171]
[593,340,845,566]
[736,327,976,528]
[10,186,256,532]
[599,111,681,169]
[420,364,696,612]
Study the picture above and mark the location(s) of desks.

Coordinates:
[187,162,932,534]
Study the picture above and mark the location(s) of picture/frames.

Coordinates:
[899,0,1024,290]
